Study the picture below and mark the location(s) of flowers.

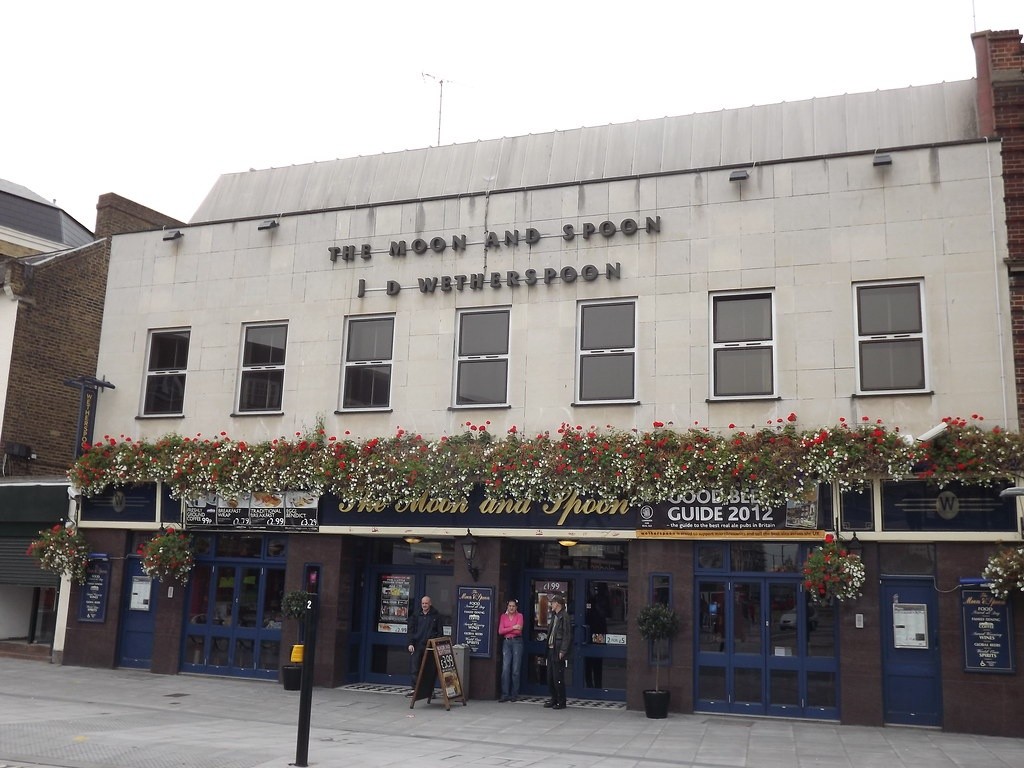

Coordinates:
[137,528,195,588]
[800,534,865,607]
[25,518,92,586]
[67,413,1024,509]
[981,539,1024,599]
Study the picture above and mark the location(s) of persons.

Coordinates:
[584,599,607,690]
[405,595,444,698]
[499,600,525,703]
[543,595,571,708]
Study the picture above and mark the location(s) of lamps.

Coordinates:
[258,212,283,230]
[873,147,892,166]
[403,536,424,544]
[461,527,479,582]
[557,539,579,546]
[163,225,184,241]
[729,161,757,181]
[847,531,864,560]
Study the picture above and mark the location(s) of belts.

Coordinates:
[505,636,521,639]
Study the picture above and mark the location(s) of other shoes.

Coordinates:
[512,696,523,702]
[499,696,509,702]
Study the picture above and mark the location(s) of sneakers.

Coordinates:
[407,692,415,697]
[553,702,566,708]
[544,698,555,707]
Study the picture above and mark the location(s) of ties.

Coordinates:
[549,616,558,644]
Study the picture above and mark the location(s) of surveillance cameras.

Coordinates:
[916,421,948,442]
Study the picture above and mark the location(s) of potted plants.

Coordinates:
[281,590,306,690]
[635,603,680,719]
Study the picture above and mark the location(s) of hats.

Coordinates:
[549,595,565,605]
